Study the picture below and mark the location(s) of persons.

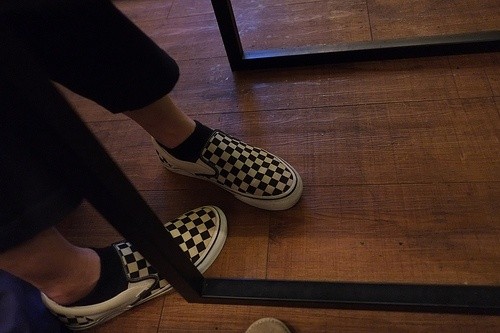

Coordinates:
[2,0,303,333]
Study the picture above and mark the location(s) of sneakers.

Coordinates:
[40,204,229,330]
[150,115,304,211]
